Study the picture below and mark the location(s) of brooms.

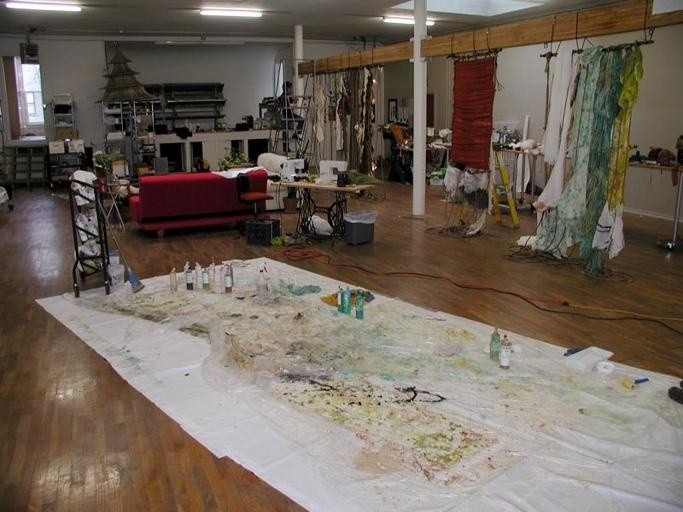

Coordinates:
[95,191,145,294]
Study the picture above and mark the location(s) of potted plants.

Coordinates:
[281,184,302,214]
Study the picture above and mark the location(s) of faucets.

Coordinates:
[215,121,229,130]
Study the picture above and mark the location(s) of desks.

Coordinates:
[537,148,682,257]
[432,141,543,218]
[389,142,449,186]
[93,179,131,236]
[270,177,376,248]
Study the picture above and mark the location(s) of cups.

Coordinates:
[595,361,614,383]
[108,256,119,265]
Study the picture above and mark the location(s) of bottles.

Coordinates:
[168,267,178,295]
[336,173,344,187]
[488,324,500,362]
[499,335,511,370]
[184,119,200,133]
[336,285,351,315]
[285,232,293,242]
[193,256,216,291]
[355,289,364,319]
[219,263,233,294]
[255,260,271,300]
[183,260,193,291]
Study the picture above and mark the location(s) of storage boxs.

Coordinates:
[429,171,446,186]
[243,217,281,247]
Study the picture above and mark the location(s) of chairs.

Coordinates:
[233,172,276,238]
[389,122,414,186]
[255,151,301,213]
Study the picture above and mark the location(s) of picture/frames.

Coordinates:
[386,97,397,122]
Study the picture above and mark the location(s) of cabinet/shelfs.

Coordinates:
[104,81,166,136]
[50,91,77,142]
[43,144,95,195]
[136,126,289,172]
[65,169,113,297]
[161,80,228,131]
[131,95,158,170]
[3,137,50,193]
[100,99,125,141]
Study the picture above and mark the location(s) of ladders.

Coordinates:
[458,144,520,229]
[269,59,322,176]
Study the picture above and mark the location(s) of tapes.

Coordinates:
[595,361,615,374]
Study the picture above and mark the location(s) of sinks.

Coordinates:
[216,129,235,132]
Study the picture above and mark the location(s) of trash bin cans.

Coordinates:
[343,209,377,246]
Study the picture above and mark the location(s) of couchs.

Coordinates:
[125,166,273,240]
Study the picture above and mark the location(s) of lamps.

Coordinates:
[3,0,82,13]
[196,7,263,18]
[381,13,436,26]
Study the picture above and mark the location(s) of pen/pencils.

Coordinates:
[635,378,650,383]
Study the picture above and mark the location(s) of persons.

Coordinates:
[278,81,304,152]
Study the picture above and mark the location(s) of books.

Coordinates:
[47,138,85,177]
[566,343,615,373]
[107,131,126,139]
